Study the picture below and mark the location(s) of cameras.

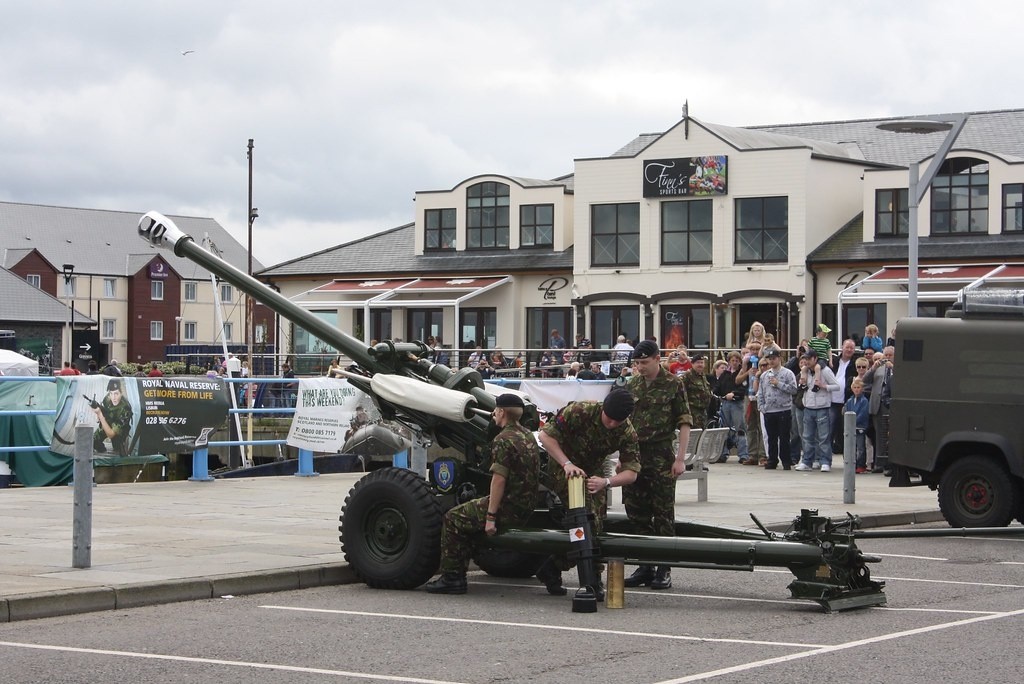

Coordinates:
[675,353,681,356]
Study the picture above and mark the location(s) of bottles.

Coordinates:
[769,371,777,388]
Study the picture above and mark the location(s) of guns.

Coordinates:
[83,393,108,429]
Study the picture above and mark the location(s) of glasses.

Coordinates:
[856,365,867,369]
[864,353,874,356]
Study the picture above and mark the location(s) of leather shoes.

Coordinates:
[651,573,671,589]
[624,568,656,588]
[425,574,467,595]
[537,564,567,596]
[586,580,604,602]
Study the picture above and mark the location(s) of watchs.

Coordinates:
[607,478,610,487]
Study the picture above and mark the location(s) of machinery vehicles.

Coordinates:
[882,286,1024,529]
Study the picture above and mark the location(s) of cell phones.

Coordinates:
[627,368,634,372]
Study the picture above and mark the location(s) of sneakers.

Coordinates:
[812,462,821,469]
[821,464,831,472]
[795,463,812,471]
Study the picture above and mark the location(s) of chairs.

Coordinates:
[607,428,727,509]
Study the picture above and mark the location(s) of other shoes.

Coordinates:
[738,458,746,464]
[758,457,768,466]
[799,384,808,391]
[716,455,727,463]
[783,465,791,470]
[855,467,868,474]
[871,466,883,473]
[765,463,777,469]
[812,385,820,392]
[743,458,758,465]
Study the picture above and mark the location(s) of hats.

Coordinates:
[815,324,832,332]
[691,354,703,364]
[497,393,524,408]
[804,349,817,358]
[107,379,120,391]
[604,389,634,423]
[764,350,780,358]
[632,340,657,360]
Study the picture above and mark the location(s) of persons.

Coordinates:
[149,361,164,377]
[93,378,132,455]
[370,341,377,346]
[465,322,895,476]
[269,364,294,408]
[541,391,641,601]
[424,396,540,596]
[327,359,340,376]
[611,343,692,588]
[133,364,148,377]
[426,335,445,360]
[345,406,369,441]
[85,359,99,375]
[103,360,123,377]
[213,352,249,407]
[59,361,82,376]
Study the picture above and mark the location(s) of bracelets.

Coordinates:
[486,512,497,522]
[563,461,571,467]
